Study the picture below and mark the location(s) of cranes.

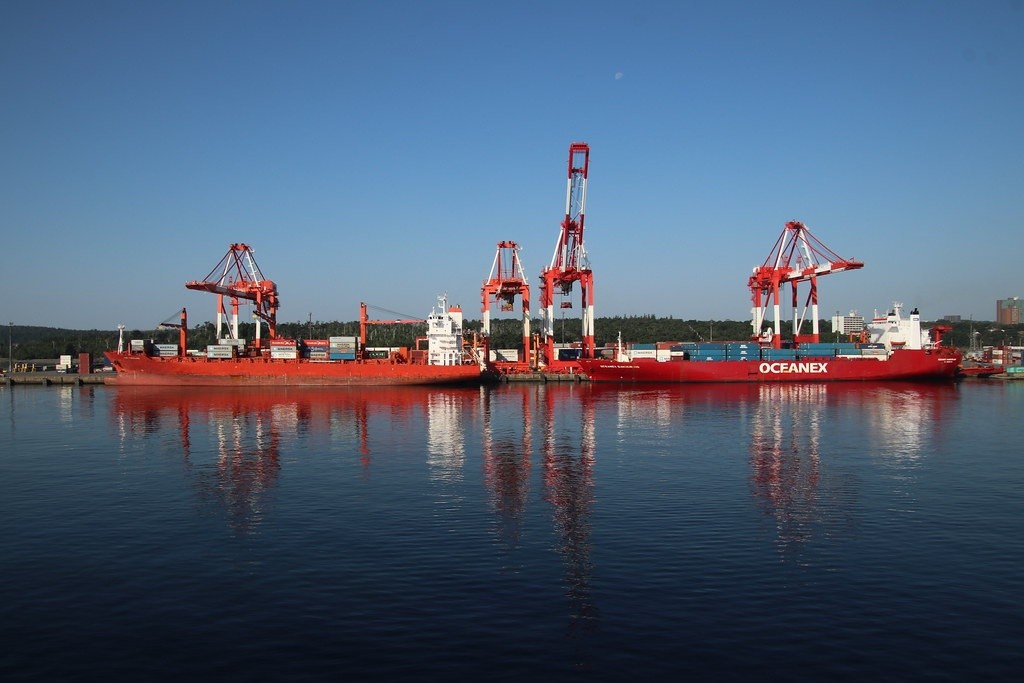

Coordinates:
[478,240,532,374]
[537,142,595,364]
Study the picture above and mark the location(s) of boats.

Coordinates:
[102,244,486,387]
[579,221,964,382]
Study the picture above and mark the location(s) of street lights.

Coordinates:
[8,321,14,371]
